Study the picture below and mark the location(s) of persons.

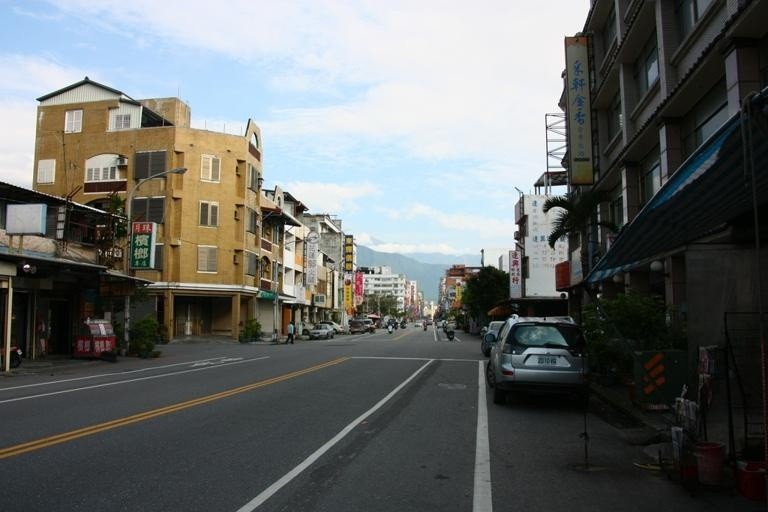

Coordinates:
[285,321,294,344]
[445,321,453,338]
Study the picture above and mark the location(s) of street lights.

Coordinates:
[125,168,186,356]
[274,237,315,342]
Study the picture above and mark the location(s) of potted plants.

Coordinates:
[580,292,672,404]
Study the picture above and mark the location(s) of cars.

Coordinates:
[302,320,344,340]
[482,313,592,405]
[349,317,433,335]
[437,320,458,329]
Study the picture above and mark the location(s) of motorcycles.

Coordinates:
[0,345,22,369]
[447,330,455,341]
